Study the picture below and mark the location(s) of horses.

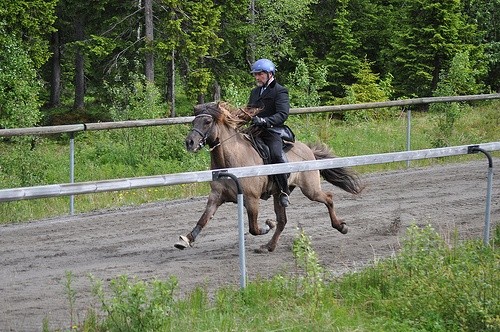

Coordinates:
[173,100,367,254]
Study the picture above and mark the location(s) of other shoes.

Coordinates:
[280,188,290,207]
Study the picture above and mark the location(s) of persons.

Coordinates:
[237,59,290,208]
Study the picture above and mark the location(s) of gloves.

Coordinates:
[252,116,266,130]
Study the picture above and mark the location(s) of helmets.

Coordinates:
[250,59,275,76]
